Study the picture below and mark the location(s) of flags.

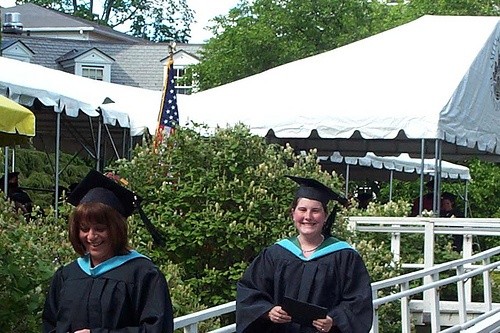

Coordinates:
[154,59,180,183]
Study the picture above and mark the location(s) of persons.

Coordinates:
[0,171,20,191]
[357,193,372,210]
[440,192,463,251]
[236,175,373,333]
[412,179,434,218]
[41,170,174,333]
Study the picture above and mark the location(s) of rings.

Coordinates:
[322,328,324,330]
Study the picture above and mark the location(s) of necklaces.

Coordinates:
[297,234,324,252]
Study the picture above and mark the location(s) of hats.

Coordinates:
[53,183,79,194]
[441,191,457,208]
[2,171,20,180]
[283,175,349,240]
[66,168,166,250]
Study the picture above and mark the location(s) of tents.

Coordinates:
[0,14,500,221]
